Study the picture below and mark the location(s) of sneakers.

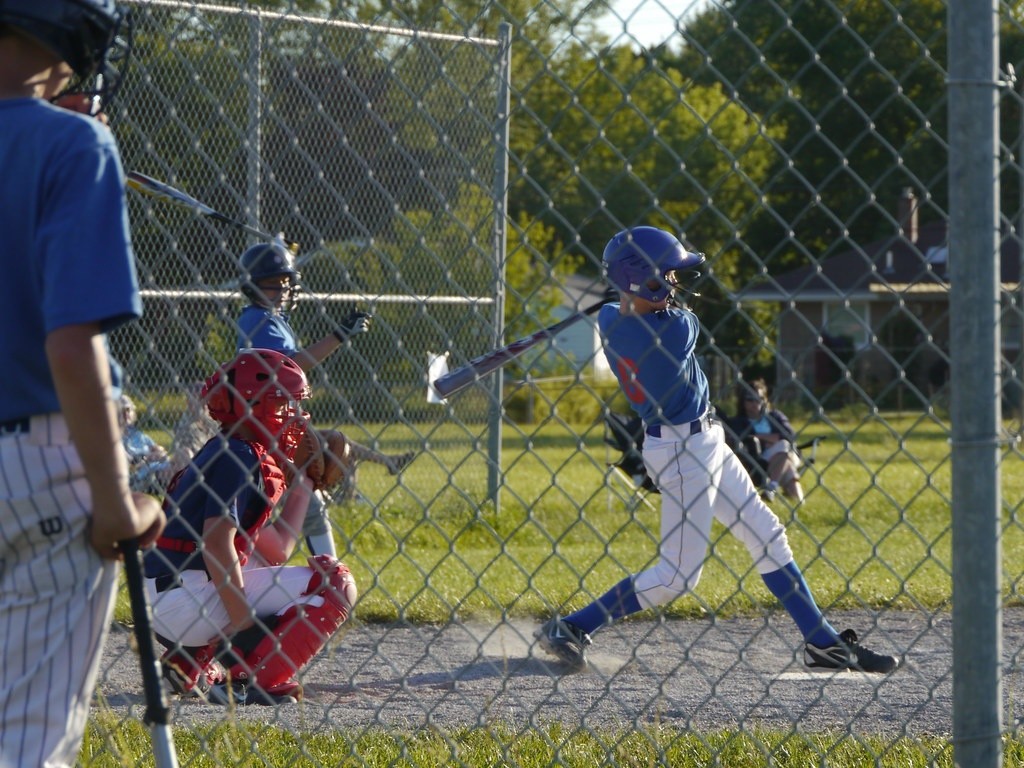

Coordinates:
[210,677,297,706]
[804,628,899,673]
[158,656,208,699]
[534,616,589,671]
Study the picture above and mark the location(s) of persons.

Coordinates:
[136,344,354,708]
[0,0,165,768]
[731,377,805,507]
[107,380,168,497]
[530,226,901,674]
[231,238,416,558]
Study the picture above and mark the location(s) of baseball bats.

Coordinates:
[127,170,300,252]
[118,536,179,768]
[433,295,618,398]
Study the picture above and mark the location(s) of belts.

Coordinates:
[156,576,183,592]
[646,419,702,437]
[3,416,30,434]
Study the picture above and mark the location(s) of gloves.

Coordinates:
[333,307,374,344]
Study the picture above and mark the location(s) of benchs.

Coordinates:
[604,409,828,511]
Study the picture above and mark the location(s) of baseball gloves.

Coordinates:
[286,427,351,491]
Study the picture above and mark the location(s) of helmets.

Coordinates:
[0,0,134,117]
[202,349,314,462]
[601,226,706,303]
[234,243,301,310]
